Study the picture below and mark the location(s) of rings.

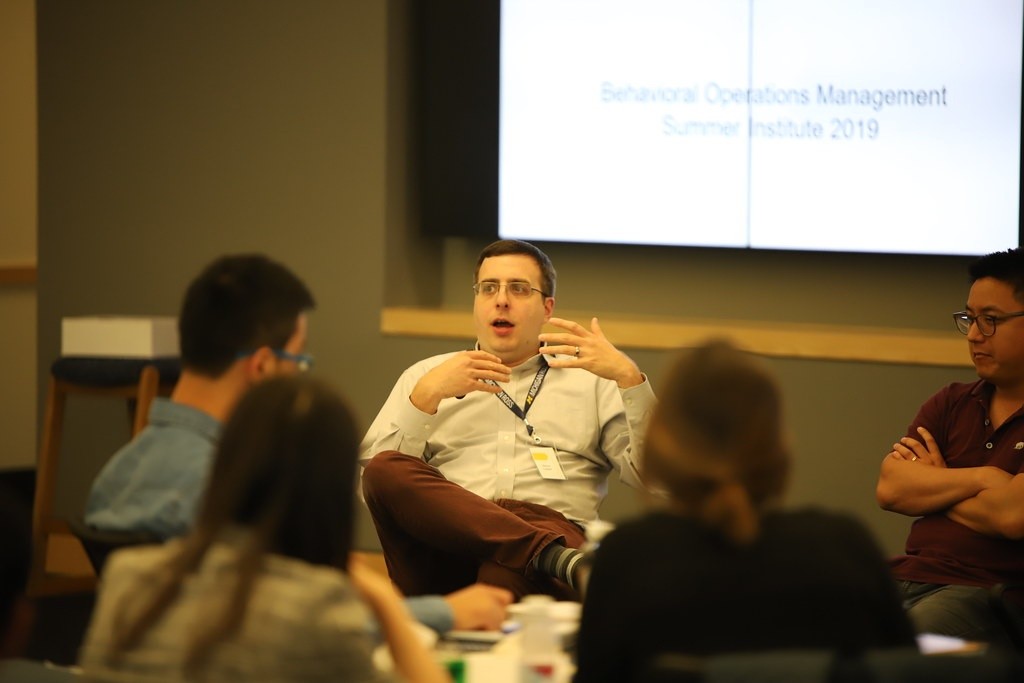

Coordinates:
[911,456,917,461]
[575,346,579,354]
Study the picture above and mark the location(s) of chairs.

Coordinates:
[65,512,166,580]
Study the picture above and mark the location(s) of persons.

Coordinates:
[355,239,658,605]
[568,335,920,683]
[873,245,1023,649]
[77,374,462,683]
[82,249,317,544]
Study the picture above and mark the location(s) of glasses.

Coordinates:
[472,282,548,298]
[953,310,1024,336]
[237,348,313,376]
